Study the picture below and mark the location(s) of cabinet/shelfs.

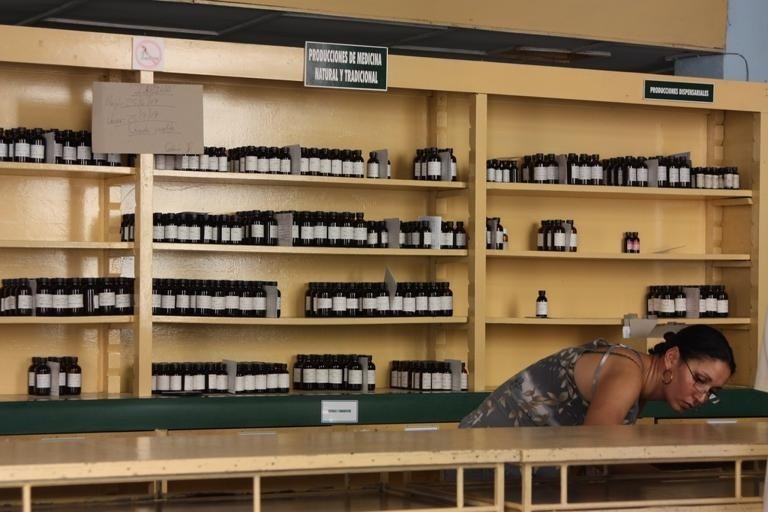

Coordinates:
[0,27,767,399]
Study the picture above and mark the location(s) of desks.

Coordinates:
[0,424,522,512]
[405,421,768,512]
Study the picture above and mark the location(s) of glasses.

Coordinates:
[682,359,721,405]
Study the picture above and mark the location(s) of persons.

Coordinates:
[445,324,736,483]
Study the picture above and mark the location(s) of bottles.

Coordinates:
[493,217,503,249]
[632,232,640,254]
[67,357,81,395]
[128,213,134,241]
[646,284,730,318]
[28,356,38,395]
[36,358,51,395]
[487,217,492,249]
[1,276,135,315]
[502,229,509,249]
[152,353,468,397]
[0,127,123,166]
[625,231,633,253]
[152,209,467,249]
[55,359,67,395]
[155,143,459,182]
[487,152,740,190]
[536,290,549,318]
[151,276,454,316]
[121,215,129,242]
[48,356,55,363]
[537,219,577,252]
